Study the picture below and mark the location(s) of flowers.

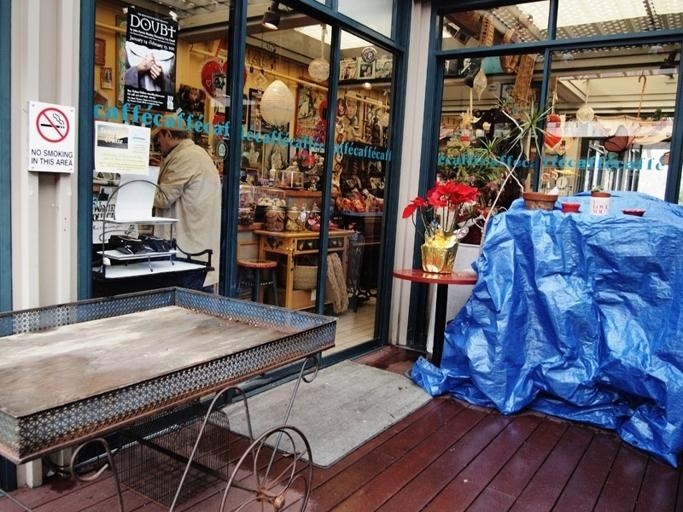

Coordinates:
[403,181,488,240]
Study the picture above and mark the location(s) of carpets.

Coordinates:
[202,359,436,470]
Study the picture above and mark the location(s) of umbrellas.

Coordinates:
[592,71,673,147]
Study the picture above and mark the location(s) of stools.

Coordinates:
[238,257,281,308]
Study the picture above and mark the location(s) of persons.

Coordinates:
[312,92,327,125]
[148,114,222,294]
[125,41,175,96]
[340,98,358,128]
[179,85,205,138]
[335,98,345,123]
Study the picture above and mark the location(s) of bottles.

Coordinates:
[591,193,610,216]
[263,206,305,232]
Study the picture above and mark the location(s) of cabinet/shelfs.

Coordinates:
[252,229,358,325]
[341,206,384,300]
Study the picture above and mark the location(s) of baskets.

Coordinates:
[277,263,318,291]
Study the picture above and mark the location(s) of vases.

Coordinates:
[420,235,460,274]
[522,191,558,211]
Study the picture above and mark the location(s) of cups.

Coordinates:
[561,203,580,213]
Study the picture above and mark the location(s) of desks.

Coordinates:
[393,269,479,366]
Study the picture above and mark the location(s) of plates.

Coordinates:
[96,249,178,260]
[623,210,644,216]
[96,215,179,227]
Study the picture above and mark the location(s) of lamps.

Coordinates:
[258,31,297,128]
[308,21,330,84]
[260,0,284,31]
[576,79,595,123]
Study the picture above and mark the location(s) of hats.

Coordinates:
[150,112,187,140]
